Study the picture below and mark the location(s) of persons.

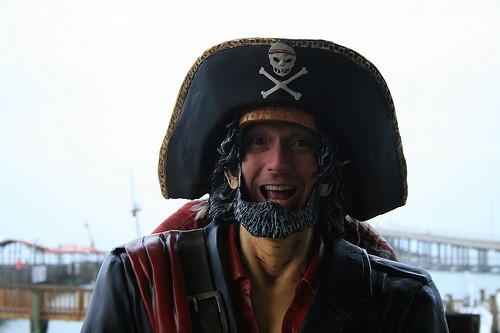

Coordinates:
[148,106,399,262]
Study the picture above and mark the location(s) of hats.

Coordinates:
[157,38,407,222]
